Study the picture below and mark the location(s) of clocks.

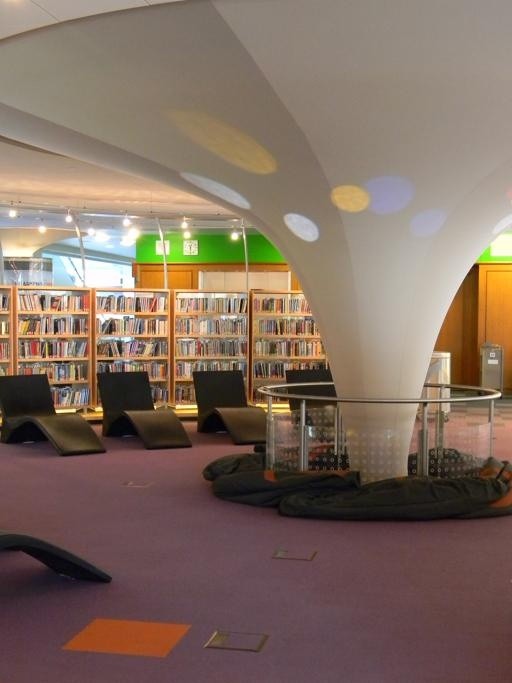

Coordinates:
[183,240,199,255]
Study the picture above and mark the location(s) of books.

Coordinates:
[93,291,170,405]
[15,288,90,407]
[0,288,11,377]
[174,291,248,405]
[251,293,331,404]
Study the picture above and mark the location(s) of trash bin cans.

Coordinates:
[418,351,451,422]
